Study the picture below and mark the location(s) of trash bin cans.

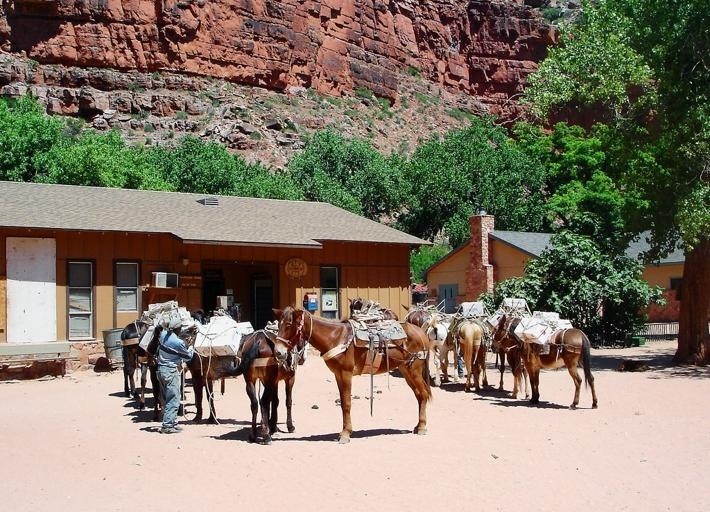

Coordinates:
[102,328,124,362]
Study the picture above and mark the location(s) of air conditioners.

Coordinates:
[152,271,178,287]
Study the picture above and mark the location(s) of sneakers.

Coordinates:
[161,426,182,433]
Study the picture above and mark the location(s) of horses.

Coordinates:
[495,316,530,400]
[491,314,597,410]
[272,303,433,444]
[120,309,204,409]
[443,320,488,392]
[401,303,432,334]
[221,331,305,445]
[347,297,398,324]
[147,324,279,425]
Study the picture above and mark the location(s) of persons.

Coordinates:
[156,317,196,434]
[457,350,475,379]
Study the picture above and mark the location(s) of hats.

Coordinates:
[169,318,182,329]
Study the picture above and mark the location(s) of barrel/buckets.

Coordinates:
[100,328,124,368]
